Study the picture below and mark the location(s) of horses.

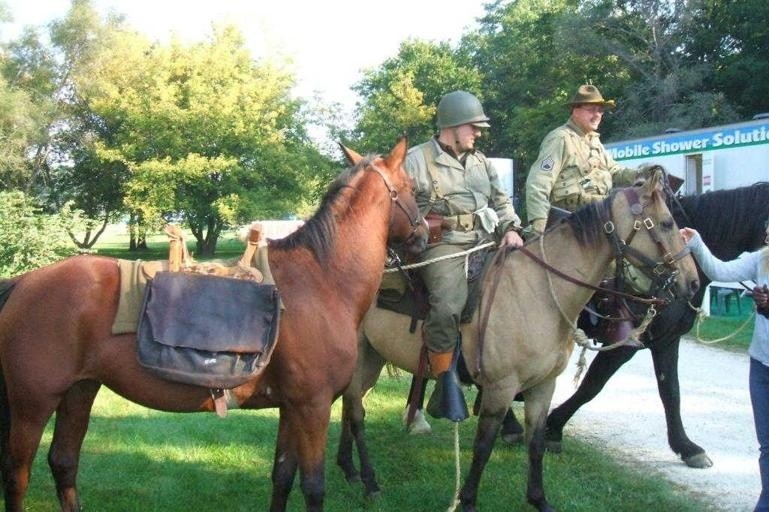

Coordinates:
[1,135,431,512]
[402,180,769,470]
[334,171,701,512]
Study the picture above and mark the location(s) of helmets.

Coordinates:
[436,91,490,128]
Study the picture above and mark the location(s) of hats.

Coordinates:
[471,121,491,128]
[561,85,616,108]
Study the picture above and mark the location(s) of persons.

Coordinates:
[522,83,660,290]
[402,91,525,372]
[677,216,768,511]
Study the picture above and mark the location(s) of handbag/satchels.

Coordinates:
[137,272,281,389]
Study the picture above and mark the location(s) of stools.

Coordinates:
[709,286,741,315]
